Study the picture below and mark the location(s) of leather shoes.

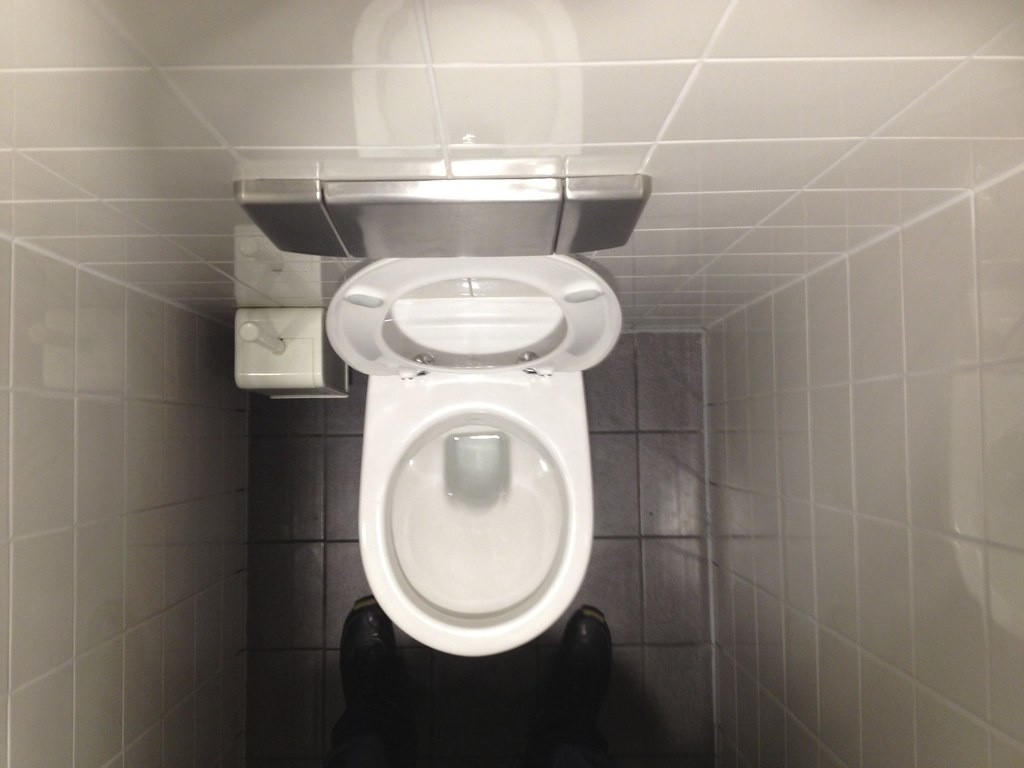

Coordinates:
[340,595,394,707]
[542,605,613,720]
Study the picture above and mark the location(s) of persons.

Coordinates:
[324,596,616,768]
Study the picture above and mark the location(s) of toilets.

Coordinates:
[231,153,652,662]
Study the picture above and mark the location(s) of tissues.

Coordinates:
[232,306,350,404]
